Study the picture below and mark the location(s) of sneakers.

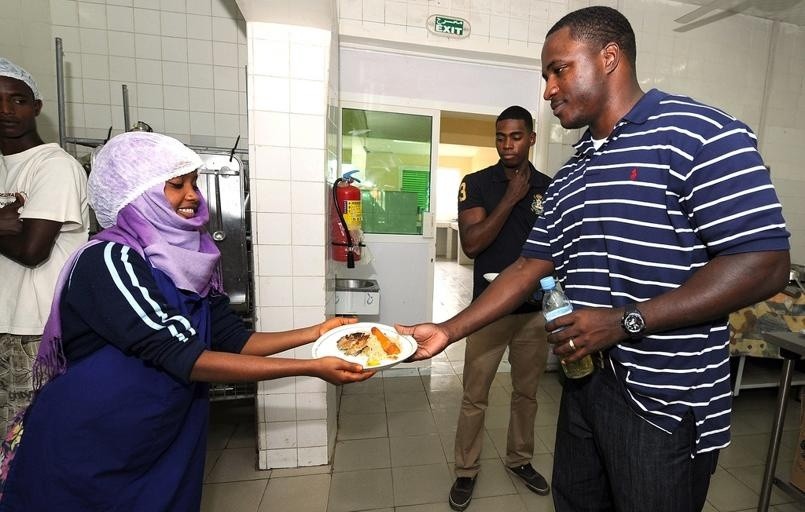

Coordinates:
[448,472,478,512]
[505,463,550,497]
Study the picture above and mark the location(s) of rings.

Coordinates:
[569,338,579,352]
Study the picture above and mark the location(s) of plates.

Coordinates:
[483,272,501,283]
[311,321,419,373]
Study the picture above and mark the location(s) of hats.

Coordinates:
[0,56,41,101]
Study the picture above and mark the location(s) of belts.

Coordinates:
[592,348,611,375]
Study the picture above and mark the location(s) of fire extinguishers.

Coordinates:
[332,170,366,269]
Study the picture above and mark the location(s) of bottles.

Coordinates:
[540,276,594,379]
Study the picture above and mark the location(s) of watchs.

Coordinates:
[621,300,649,340]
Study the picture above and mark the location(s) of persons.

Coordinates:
[446,105,564,512]
[0,54,89,439]
[0,128,374,511]
[394,5,791,512]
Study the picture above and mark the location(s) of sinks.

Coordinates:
[333,277,380,295]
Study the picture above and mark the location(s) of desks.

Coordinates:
[756,324,804,512]
